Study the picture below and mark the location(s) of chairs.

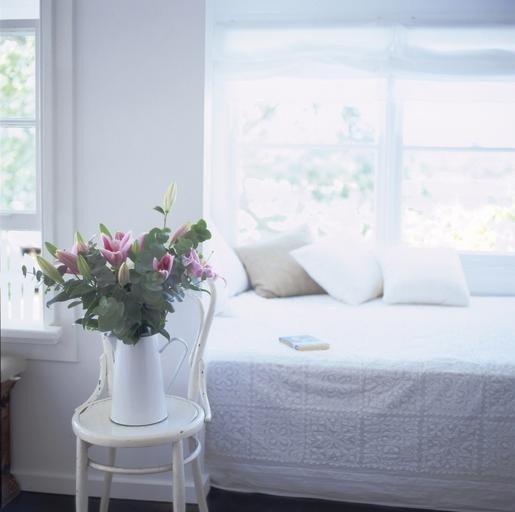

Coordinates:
[185,252,515,511]
[71,277,217,511]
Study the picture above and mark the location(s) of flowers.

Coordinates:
[22,179,229,346]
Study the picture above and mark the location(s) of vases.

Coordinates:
[109,331,168,428]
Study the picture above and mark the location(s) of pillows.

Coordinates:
[233,220,471,307]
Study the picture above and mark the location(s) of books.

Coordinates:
[279,336,330,351]
